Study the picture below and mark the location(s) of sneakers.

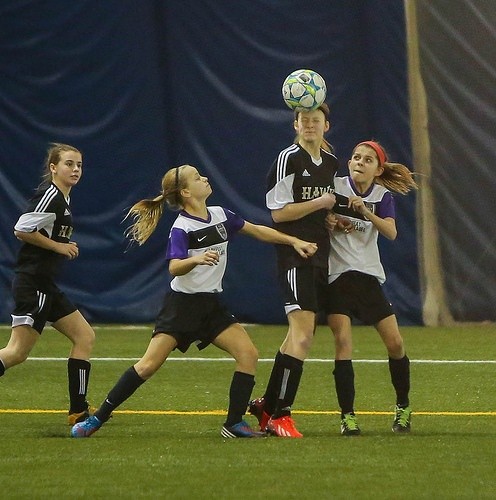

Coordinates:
[341,412,360,436]
[249,398,270,431]
[391,403,412,433]
[221,421,267,438]
[67,404,113,426]
[70,416,102,437]
[266,415,303,437]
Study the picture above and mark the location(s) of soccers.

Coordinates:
[282,68,327,114]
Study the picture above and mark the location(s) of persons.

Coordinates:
[326,140,431,436]
[0,142,114,427]
[70,165,318,438]
[247,103,339,438]
